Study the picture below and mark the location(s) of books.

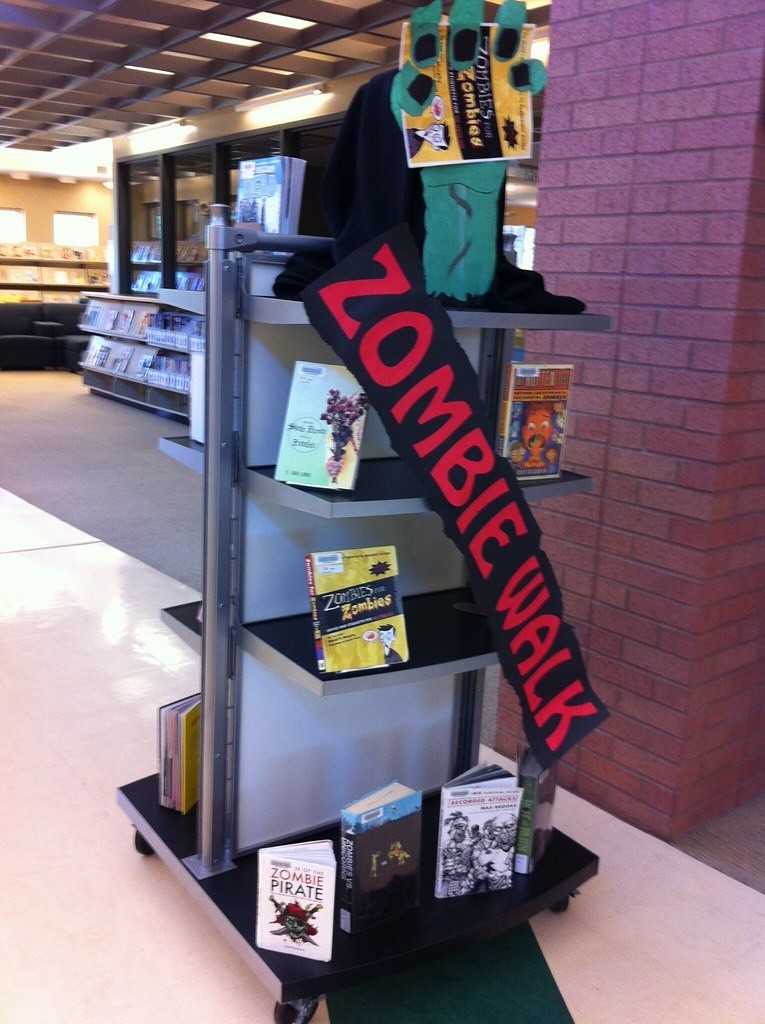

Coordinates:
[304,545,410,675]
[254,839,337,963]
[337,777,422,934]
[434,743,557,899]
[153,691,203,816]
[234,156,307,256]
[273,359,369,492]
[0,243,206,379]
[495,361,575,480]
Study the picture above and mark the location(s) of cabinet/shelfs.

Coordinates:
[116,204,611,1024]
[114,110,349,296]
[77,292,205,422]
[0,244,108,303]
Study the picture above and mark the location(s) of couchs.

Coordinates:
[0,303,66,370]
[41,303,91,374]
